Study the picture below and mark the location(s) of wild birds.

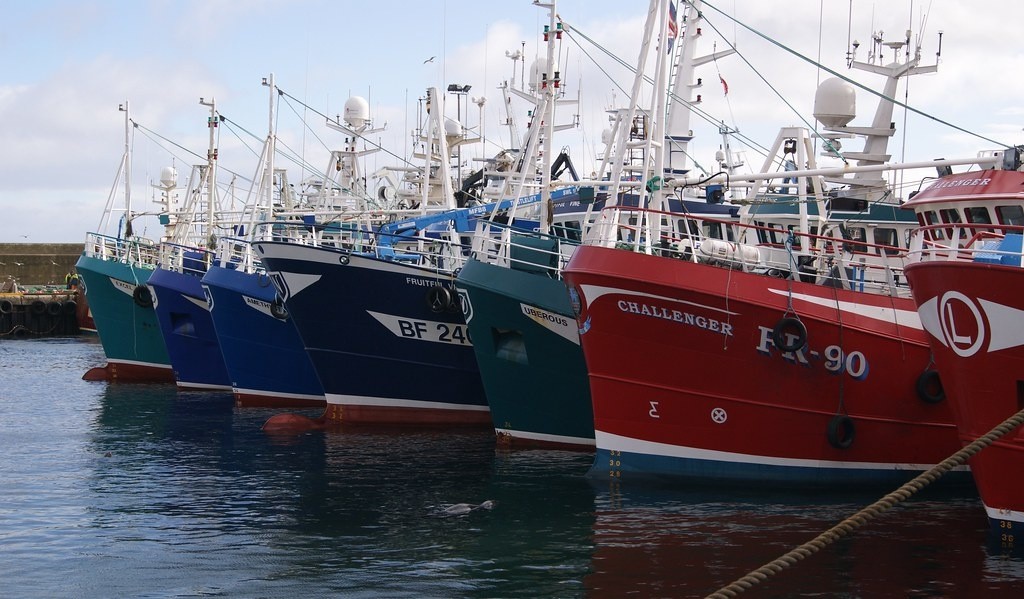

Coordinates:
[424,56,434,64]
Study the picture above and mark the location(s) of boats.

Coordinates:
[559,0,976,491]
[899,143,1024,555]
[74,0,945,456]
[0,274,99,340]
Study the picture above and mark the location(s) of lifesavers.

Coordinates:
[916,370,947,406]
[133,285,154,308]
[425,286,461,313]
[270,298,291,320]
[828,413,855,449]
[773,318,807,353]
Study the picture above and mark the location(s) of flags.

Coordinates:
[656,2,678,55]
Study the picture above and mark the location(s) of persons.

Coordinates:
[64,269,78,289]
